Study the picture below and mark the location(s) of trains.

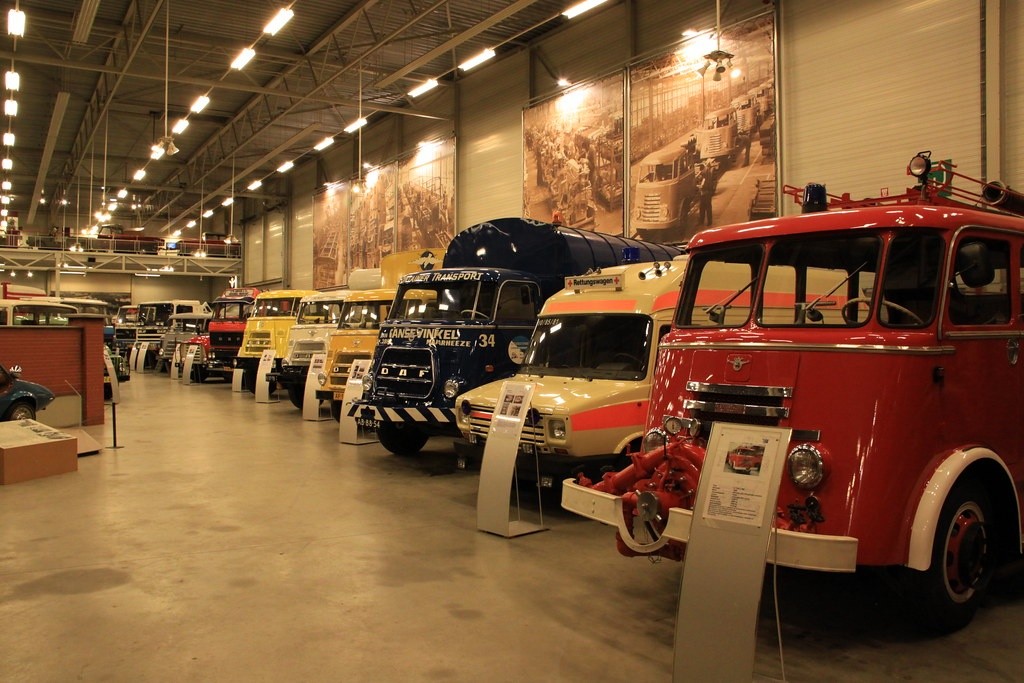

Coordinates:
[635,72,775,241]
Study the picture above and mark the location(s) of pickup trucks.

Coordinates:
[96,223,165,250]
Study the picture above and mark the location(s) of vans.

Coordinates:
[0,281,130,400]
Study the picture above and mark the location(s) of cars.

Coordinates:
[0,365,57,421]
[112,287,438,431]
[178,233,243,256]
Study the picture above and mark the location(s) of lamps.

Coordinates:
[697,0,741,82]
[153,0,180,157]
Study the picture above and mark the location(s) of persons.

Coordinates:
[696,164,713,226]
[737,123,749,167]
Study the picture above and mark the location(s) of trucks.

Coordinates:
[347,152,1024,637]
